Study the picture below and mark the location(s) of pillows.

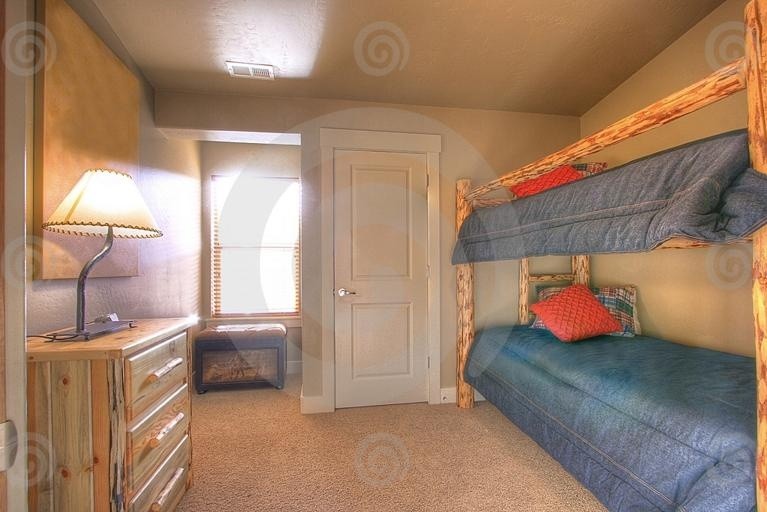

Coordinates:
[526,283,642,344]
[508,161,608,200]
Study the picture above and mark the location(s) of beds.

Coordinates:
[449,0,767,512]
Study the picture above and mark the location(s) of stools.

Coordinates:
[193,323,287,394]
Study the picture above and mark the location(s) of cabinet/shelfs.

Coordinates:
[26,316,199,511]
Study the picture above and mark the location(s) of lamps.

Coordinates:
[43,170,163,343]
